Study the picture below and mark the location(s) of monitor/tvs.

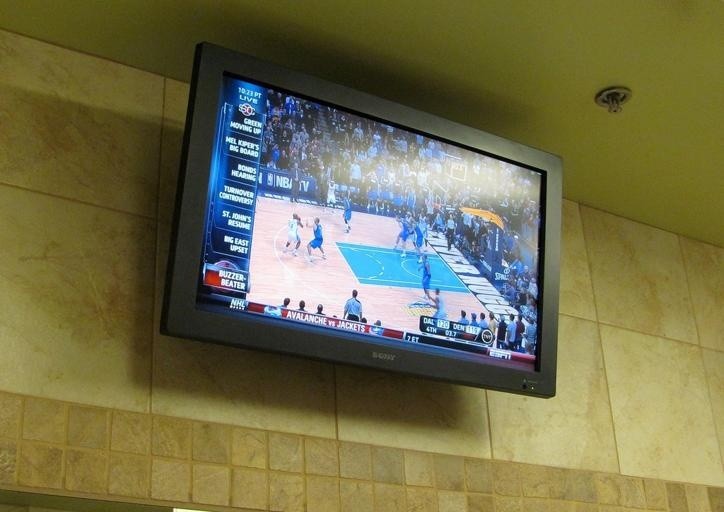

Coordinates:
[159,41,563,398]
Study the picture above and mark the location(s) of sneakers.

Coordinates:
[421,293,429,300]
[393,243,424,264]
[282,247,327,262]
[343,223,351,233]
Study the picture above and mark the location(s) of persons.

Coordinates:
[261,84,540,355]
